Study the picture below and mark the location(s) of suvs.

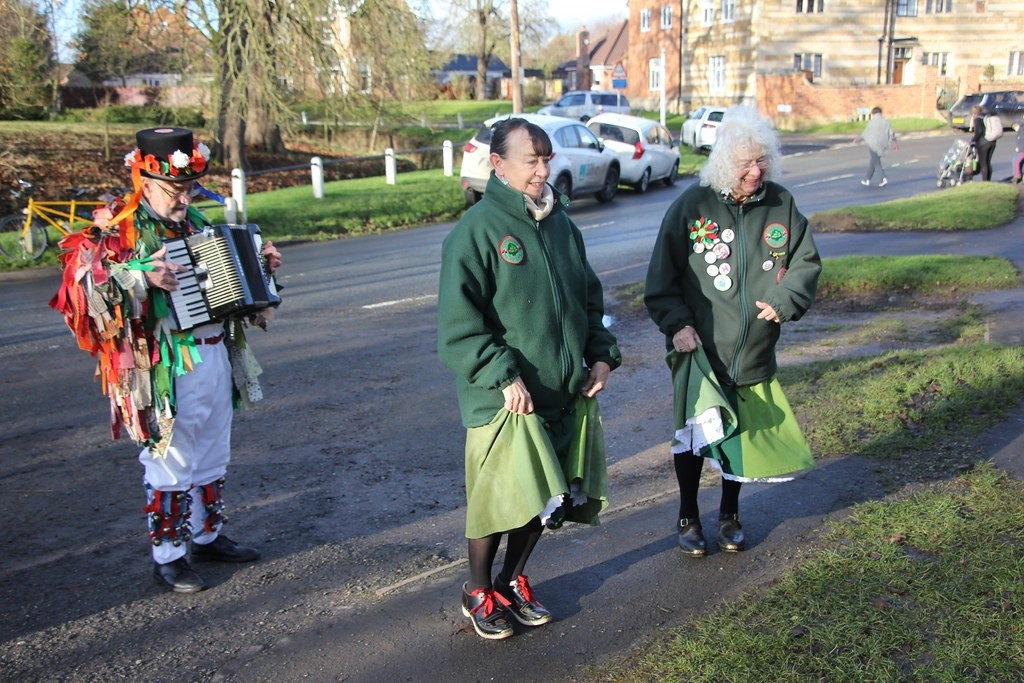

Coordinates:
[537,91,630,123]
[459,113,621,206]
[948,90,1024,133]
[585,112,681,193]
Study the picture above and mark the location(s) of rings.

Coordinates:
[679,347,685,351]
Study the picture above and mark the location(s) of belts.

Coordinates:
[194,331,224,345]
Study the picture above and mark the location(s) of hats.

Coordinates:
[123,127,210,182]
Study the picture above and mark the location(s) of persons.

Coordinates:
[642,103,822,559]
[852,107,899,187]
[1011,120,1024,183]
[438,119,622,519]
[969,105,995,183]
[57,127,282,593]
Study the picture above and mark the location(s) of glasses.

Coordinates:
[156,184,201,201]
[740,156,770,172]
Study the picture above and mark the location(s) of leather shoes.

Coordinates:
[676,518,706,557]
[190,535,260,563]
[154,559,208,594]
[717,512,745,552]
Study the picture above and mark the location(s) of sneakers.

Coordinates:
[461,582,515,640]
[494,573,552,624]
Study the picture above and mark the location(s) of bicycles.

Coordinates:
[0,179,108,262]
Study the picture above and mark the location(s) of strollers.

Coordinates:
[936,139,981,188]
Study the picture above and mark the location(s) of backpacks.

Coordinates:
[983,112,1003,142]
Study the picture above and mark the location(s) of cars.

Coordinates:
[680,106,726,153]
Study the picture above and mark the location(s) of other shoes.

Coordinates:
[861,179,869,185]
[878,178,888,188]
